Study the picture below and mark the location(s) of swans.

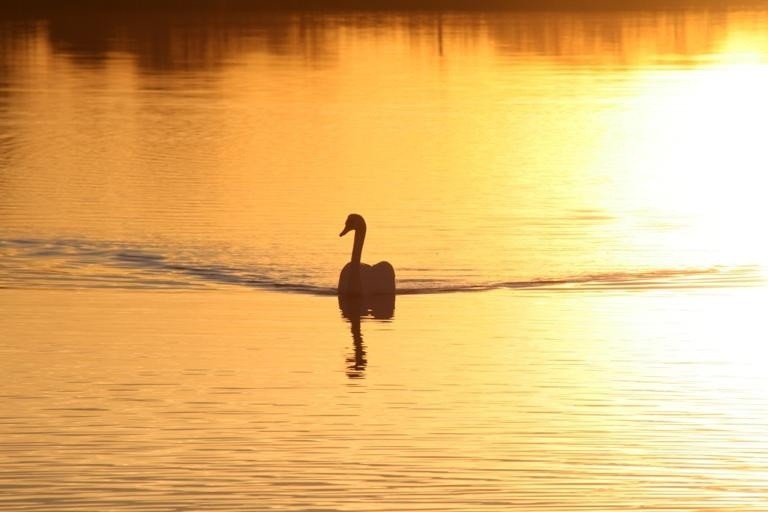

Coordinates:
[337,213,396,319]
